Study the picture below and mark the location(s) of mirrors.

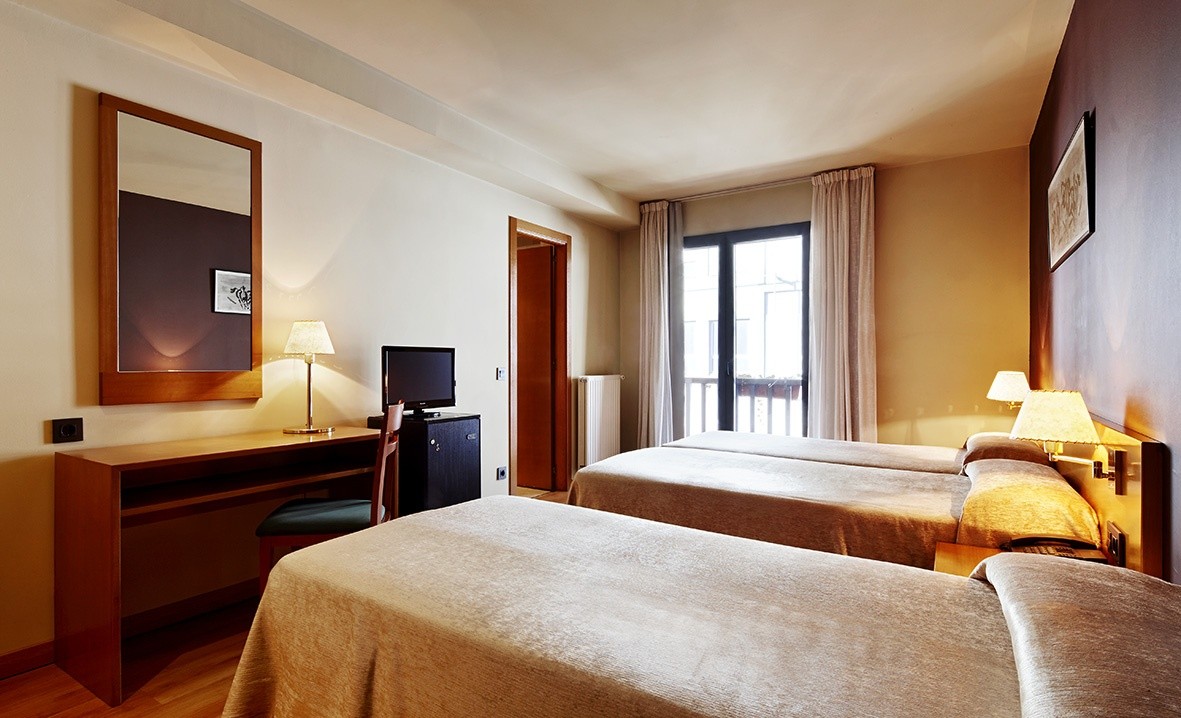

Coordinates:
[96,91,264,410]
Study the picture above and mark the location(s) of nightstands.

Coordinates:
[933,539,1004,577]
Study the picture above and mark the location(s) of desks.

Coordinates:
[51,426,382,707]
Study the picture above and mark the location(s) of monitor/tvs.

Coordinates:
[382,345,455,418]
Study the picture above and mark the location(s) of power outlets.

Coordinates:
[1106,519,1125,565]
[52,417,84,445]
[497,467,507,481]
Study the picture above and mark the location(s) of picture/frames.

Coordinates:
[213,267,251,316]
[1045,111,1095,274]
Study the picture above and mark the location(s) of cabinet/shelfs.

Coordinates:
[366,412,484,518]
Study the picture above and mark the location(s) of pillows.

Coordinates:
[969,550,1181,718]
[960,431,1049,476]
[957,459,1105,548]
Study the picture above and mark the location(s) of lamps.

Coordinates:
[1010,388,1123,497]
[986,370,1030,410]
[283,320,336,435]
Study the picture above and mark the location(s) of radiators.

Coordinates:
[577,375,626,470]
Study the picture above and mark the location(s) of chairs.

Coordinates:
[255,399,405,599]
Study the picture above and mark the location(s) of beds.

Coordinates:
[663,430,1050,477]
[220,494,1025,718]
[566,412,1170,577]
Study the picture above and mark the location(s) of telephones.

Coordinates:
[1008,533,1108,564]
